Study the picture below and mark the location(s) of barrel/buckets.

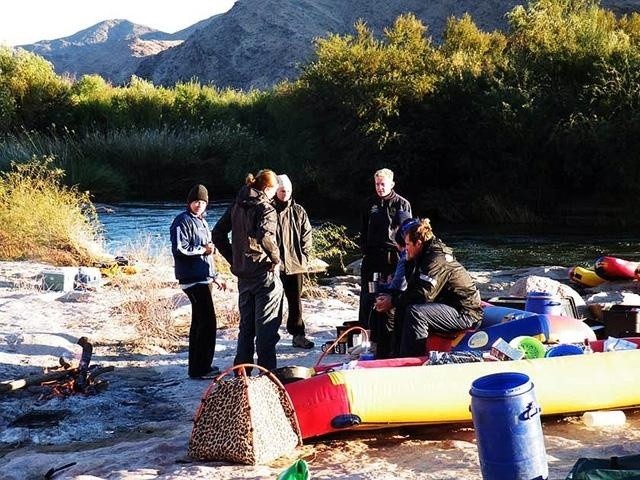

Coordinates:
[525,292,561,315]
[468,373,548,479]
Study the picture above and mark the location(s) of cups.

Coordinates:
[323,341,347,354]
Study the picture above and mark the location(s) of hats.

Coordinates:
[187,184,208,203]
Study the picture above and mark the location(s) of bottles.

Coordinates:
[583,337,594,355]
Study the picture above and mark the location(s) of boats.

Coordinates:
[264,341,640,438]
[597,253,640,282]
[573,265,601,288]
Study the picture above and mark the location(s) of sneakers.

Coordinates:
[201,370,221,379]
[293,335,314,349]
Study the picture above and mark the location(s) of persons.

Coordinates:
[267,173,315,351]
[360,167,411,328]
[372,216,485,357]
[358,220,418,360]
[212,168,283,377]
[169,182,231,380]
